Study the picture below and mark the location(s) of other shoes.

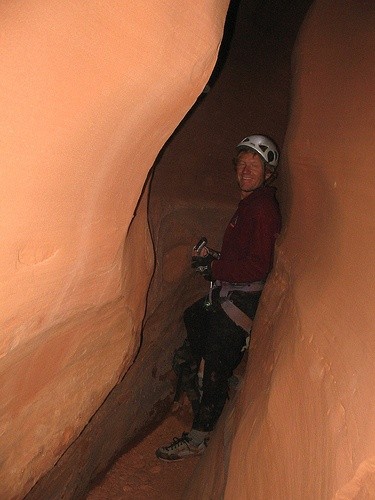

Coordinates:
[157,433,209,461]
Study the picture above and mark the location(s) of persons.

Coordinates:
[156,135,281,461]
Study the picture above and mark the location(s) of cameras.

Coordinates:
[194,239,207,253]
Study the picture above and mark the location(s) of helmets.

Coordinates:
[234,134,280,166]
